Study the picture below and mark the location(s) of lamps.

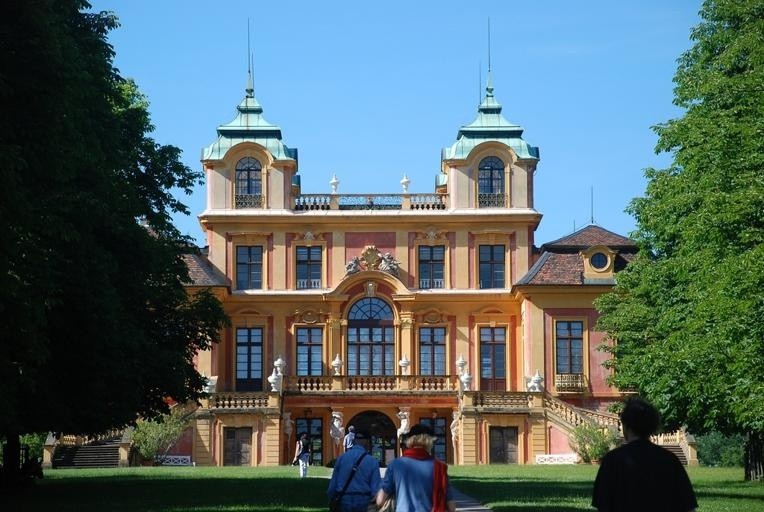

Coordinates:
[304,408,312,419]
[430,409,440,420]
[363,280,378,298]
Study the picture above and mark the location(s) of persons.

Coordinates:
[326,435,381,510]
[591,398,698,511]
[389,428,397,460]
[341,425,356,453]
[375,423,456,512]
[292,432,312,478]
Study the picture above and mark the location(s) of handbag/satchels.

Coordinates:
[329,492,342,511]
[369,492,396,512]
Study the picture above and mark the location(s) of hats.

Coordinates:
[355,428,372,440]
[408,424,436,436]
